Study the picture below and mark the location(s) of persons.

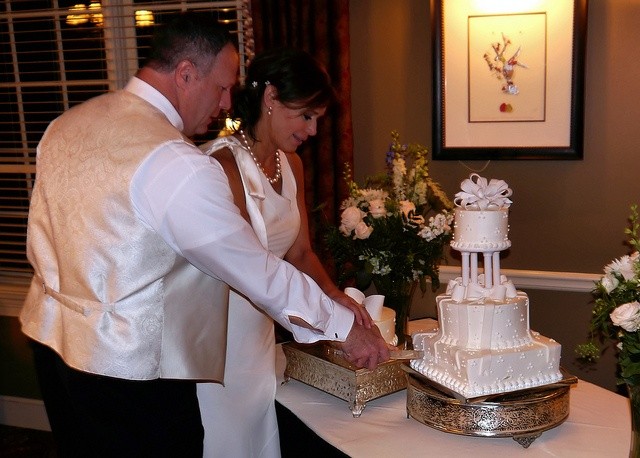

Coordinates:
[197,46,376,458]
[19,13,398,457]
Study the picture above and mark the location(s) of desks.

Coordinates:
[272,341,631,457]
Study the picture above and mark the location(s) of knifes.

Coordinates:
[327,349,426,360]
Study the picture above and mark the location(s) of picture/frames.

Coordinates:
[427,0,587,164]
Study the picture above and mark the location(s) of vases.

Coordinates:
[627,385,640,458]
[373,275,414,349]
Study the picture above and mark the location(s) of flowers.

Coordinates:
[317,126,456,298]
[572,201,640,386]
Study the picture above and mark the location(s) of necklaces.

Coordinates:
[239,129,282,184]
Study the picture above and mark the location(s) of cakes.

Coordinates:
[410,172,563,399]
[344,287,398,346]
